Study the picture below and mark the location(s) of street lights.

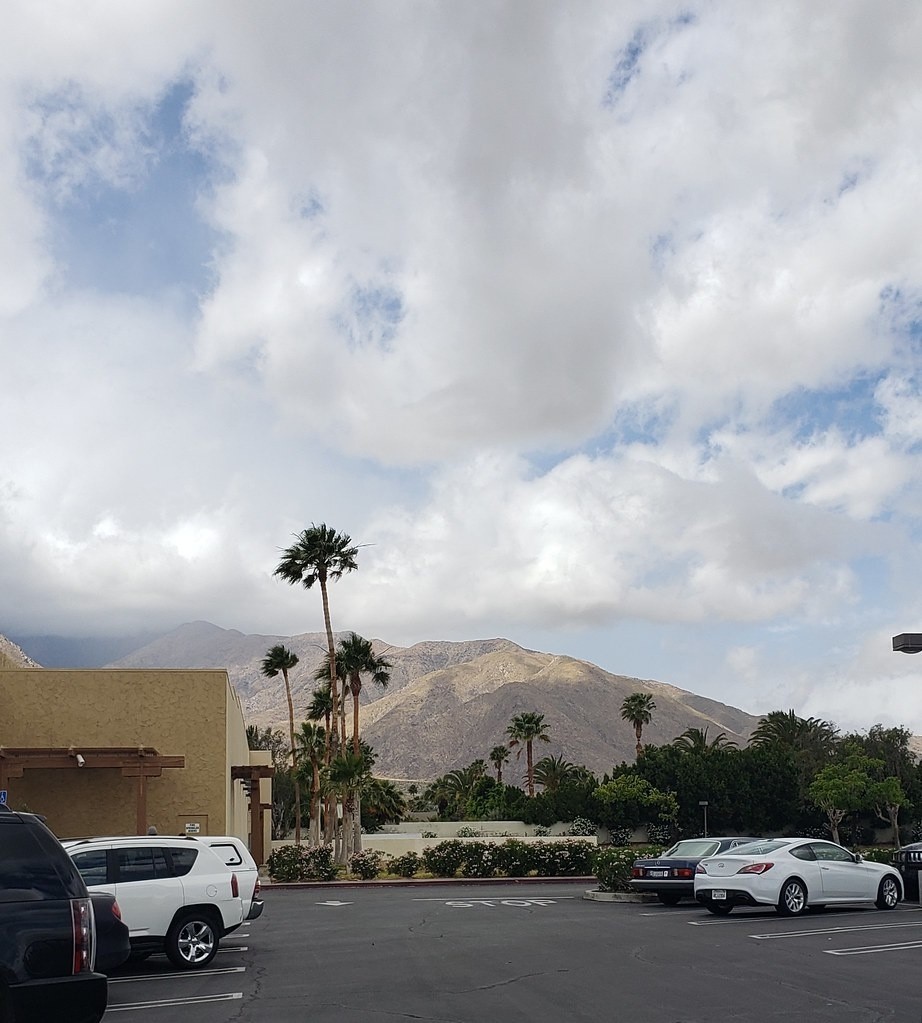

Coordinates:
[699,801,708,837]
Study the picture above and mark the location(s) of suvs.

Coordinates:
[0,803,108,1023]
[56,835,243,970]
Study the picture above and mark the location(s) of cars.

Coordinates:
[629,838,759,907]
[694,838,905,917]
[73,835,265,920]
